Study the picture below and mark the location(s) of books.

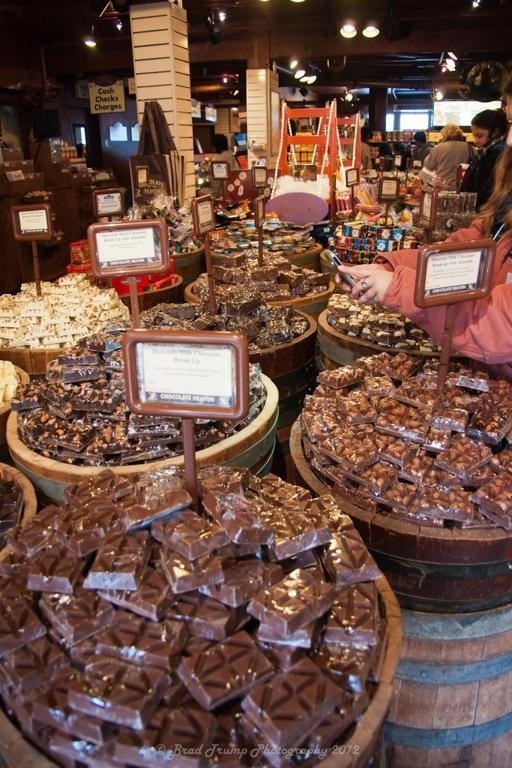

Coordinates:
[128,150,186,208]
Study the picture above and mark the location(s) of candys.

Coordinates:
[0,196,512,768]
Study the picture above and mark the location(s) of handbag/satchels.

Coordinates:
[129,101,186,211]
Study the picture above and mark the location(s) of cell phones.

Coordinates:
[321,247,362,290]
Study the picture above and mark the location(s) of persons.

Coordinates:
[427,123,477,192]
[335,73,512,379]
[346,127,374,170]
[413,130,434,161]
[461,107,507,240]
[209,144,217,152]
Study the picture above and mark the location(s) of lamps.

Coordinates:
[439,37,471,72]
[290,58,322,86]
[339,14,383,40]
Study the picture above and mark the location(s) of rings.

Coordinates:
[360,280,370,289]
